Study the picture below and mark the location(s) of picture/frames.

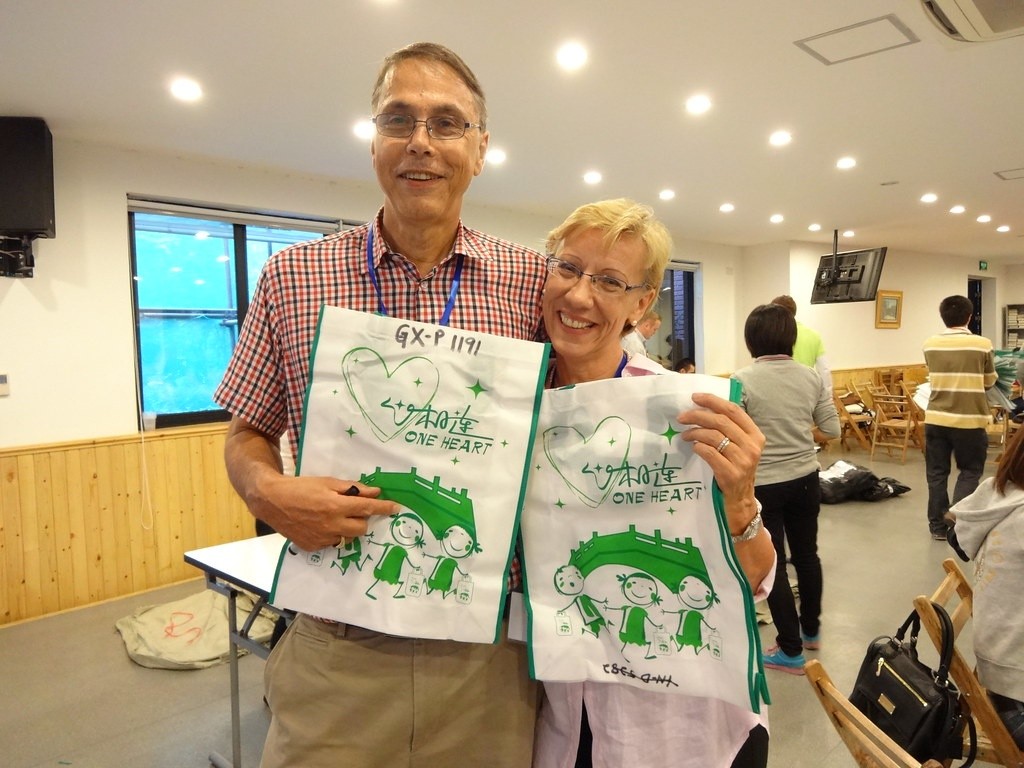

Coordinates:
[876,289,903,330]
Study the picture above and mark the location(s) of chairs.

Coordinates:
[1002,409,1021,452]
[803,557,1024,767]
[831,369,926,464]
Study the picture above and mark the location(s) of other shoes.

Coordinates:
[929,526,953,540]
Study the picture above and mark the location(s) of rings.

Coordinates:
[333,536,345,550]
[717,437,730,453]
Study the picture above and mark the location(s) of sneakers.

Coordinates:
[801,633,820,650]
[762,647,806,676]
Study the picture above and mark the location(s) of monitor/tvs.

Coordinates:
[811,246,887,304]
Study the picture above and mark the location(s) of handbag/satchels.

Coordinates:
[925,348,1024,414]
[265,304,552,647]
[848,602,977,768]
[515,373,774,716]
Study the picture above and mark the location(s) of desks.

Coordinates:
[183,531,298,767]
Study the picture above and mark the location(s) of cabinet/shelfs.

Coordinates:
[1004,303,1024,351]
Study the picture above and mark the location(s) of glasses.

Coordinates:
[545,255,644,298]
[373,112,480,140]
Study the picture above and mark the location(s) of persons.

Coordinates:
[526,198,778,768]
[620,311,696,375]
[770,295,835,400]
[924,296,998,541]
[214,40,548,768]
[730,304,841,674]
[947,421,1024,756]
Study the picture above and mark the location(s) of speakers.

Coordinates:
[0,116,55,239]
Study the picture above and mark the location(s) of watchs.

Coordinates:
[731,498,763,544]
